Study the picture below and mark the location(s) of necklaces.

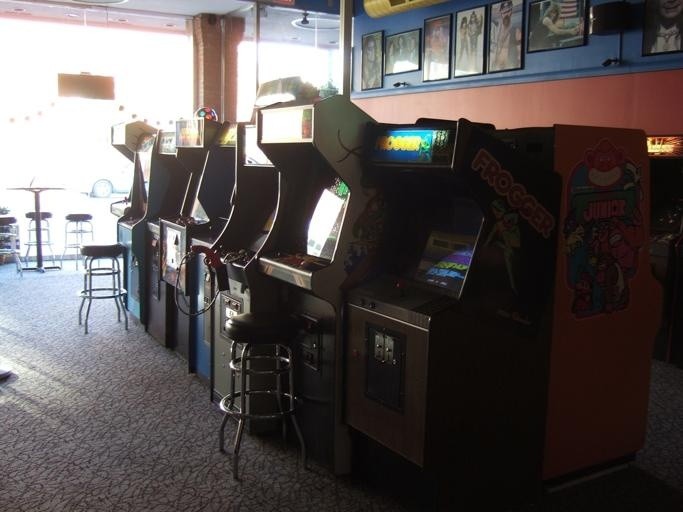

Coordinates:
[655,31,679,53]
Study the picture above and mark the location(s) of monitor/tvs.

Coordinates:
[143,181,149,203]
[189,171,209,221]
[259,206,275,234]
[416,232,476,292]
[291,164,350,262]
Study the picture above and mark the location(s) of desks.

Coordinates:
[7,184,69,273]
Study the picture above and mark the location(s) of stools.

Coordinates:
[22,209,56,268]
[74,239,132,337]
[59,213,99,273]
[0,215,22,276]
[215,310,312,482]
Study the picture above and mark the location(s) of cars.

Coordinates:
[75,170,151,199]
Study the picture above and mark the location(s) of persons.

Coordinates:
[428,19,447,54]
[396,34,407,60]
[407,36,418,65]
[384,39,395,64]
[456,15,470,66]
[361,35,380,89]
[527,1,581,49]
[467,9,483,65]
[491,0,522,69]
[642,1,682,56]
